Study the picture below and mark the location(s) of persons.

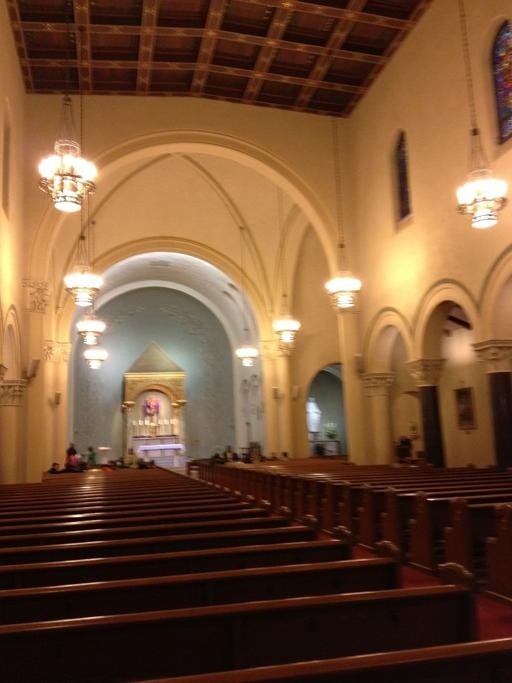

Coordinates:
[48,444,156,475]
[212,446,289,462]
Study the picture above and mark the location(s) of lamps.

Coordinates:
[238,189,302,374]
[65,210,112,369]
[38,3,98,214]
[322,93,361,311]
[456,0,509,228]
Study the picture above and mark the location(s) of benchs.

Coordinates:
[0,453,510,682]
